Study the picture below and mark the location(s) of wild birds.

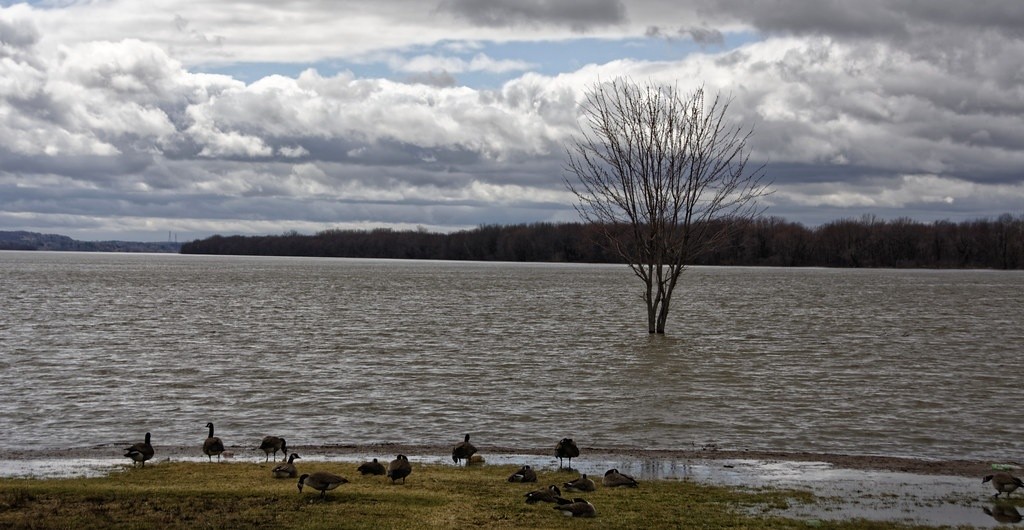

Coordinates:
[980,473,1024,499]
[297,472,349,500]
[521,473,597,519]
[601,468,640,490]
[357,459,386,476]
[123,431,155,468]
[451,434,478,466]
[270,453,301,479]
[508,465,536,484]
[981,503,1024,523]
[258,435,287,462]
[202,422,226,464]
[387,454,411,485]
[553,438,580,470]
